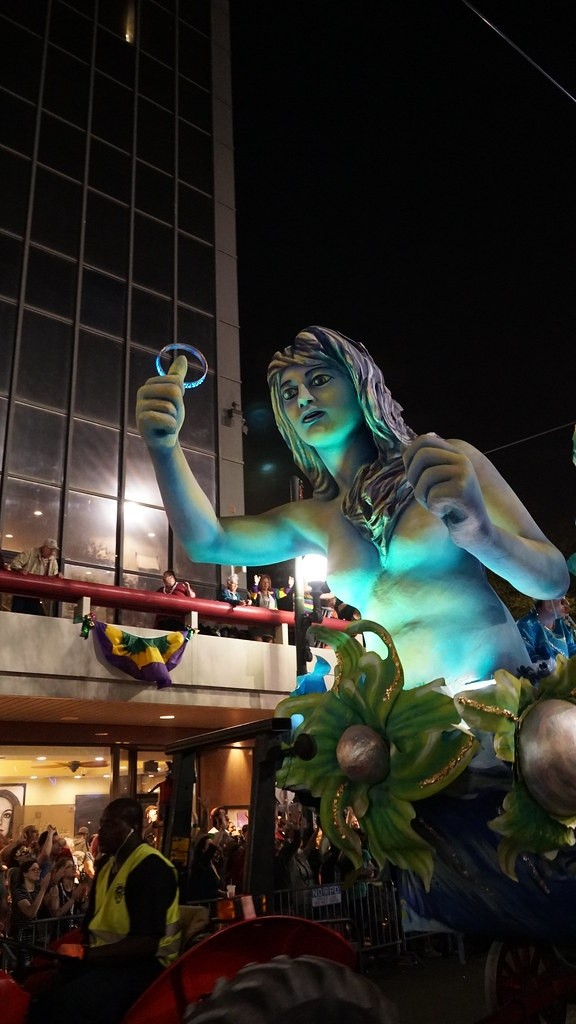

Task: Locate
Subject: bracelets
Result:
[156,343,208,387]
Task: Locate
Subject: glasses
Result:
[65,865,76,869]
[28,869,42,873]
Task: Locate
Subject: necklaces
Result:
[162,582,178,595]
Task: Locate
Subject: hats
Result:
[289,804,301,814]
[43,538,60,550]
[210,807,218,819]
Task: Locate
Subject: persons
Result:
[219,572,361,648]
[0,806,454,983]
[154,569,196,631]
[134,327,576,820]
[9,538,64,616]
[515,596,576,686]
[23,797,181,1024]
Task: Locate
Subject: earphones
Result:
[127,828,135,836]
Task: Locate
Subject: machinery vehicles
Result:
[0,714,402,1024]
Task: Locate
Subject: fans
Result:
[30,761,109,772]
